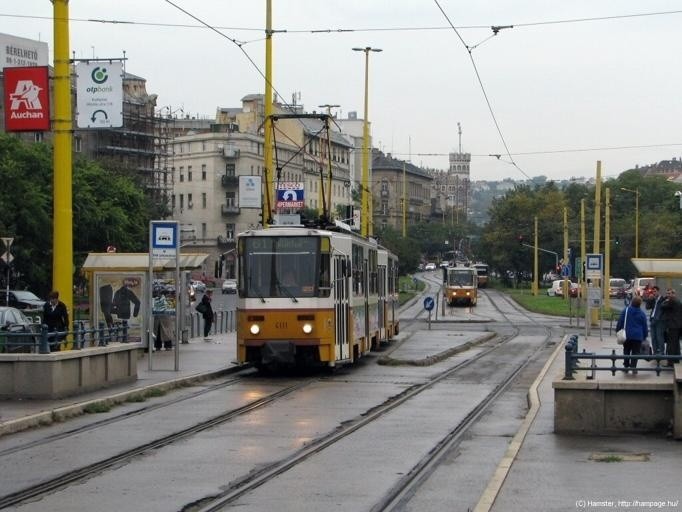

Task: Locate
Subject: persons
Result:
[153,314,172,351]
[195,289,214,339]
[43,292,69,352]
[616,284,682,374]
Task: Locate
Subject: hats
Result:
[48,291,59,298]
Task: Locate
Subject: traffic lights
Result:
[517,233,524,245]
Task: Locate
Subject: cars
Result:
[0,306,39,352]
[502,267,563,282]
[417,260,450,272]
[0,289,47,313]
[150,277,237,311]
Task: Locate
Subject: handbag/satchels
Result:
[195,301,208,313]
[639,340,653,362]
[616,329,627,344]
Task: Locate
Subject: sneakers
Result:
[204,336,212,340]
[621,367,638,374]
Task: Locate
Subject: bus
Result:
[441,266,478,308]
[445,251,462,265]
[468,263,491,288]
[229,218,402,373]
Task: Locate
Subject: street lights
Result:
[619,183,640,279]
[350,46,385,239]
[317,100,341,221]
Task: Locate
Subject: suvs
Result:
[542,276,656,301]
